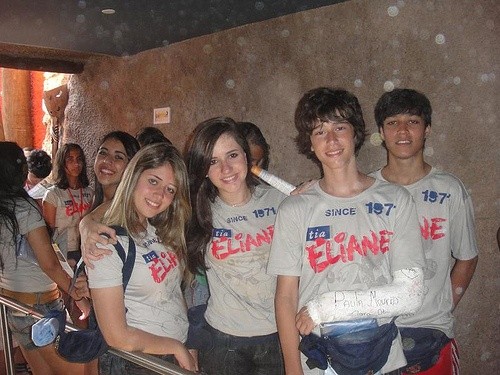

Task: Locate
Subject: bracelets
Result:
[74,296,84,302]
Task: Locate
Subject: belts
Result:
[0,289,59,304]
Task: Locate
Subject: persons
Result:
[0,141,99,374]
[370,88,480,375]
[43,143,96,277]
[83,143,200,375]
[235,121,268,176]
[134,125,171,149]
[23,147,52,197]
[71,127,139,301]
[266,86,424,374]
[79,119,323,375]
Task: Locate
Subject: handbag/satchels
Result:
[299,316,399,375]
[393,325,449,372]
[187,303,209,342]
[55,326,110,363]
